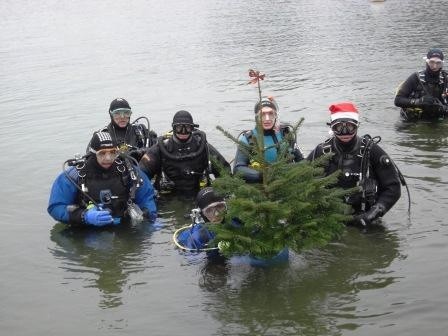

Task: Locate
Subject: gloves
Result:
[433,97,444,112]
[352,203,385,229]
[422,95,433,106]
[84,207,114,226]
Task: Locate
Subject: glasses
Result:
[88,147,117,160]
[201,202,227,217]
[110,110,132,118]
[332,123,357,135]
[427,59,444,67]
[173,124,194,134]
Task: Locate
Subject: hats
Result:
[254,96,279,114]
[427,49,444,61]
[89,130,117,151]
[326,102,359,128]
[109,98,132,113]
[196,187,224,210]
[172,110,194,127]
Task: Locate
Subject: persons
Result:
[47,129,165,250]
[306,103,410,235]
[139,111,232,202]
[394,48,448,132]
[234,96,303,183]
[86,98,163,205]
[175,187,288,292]
